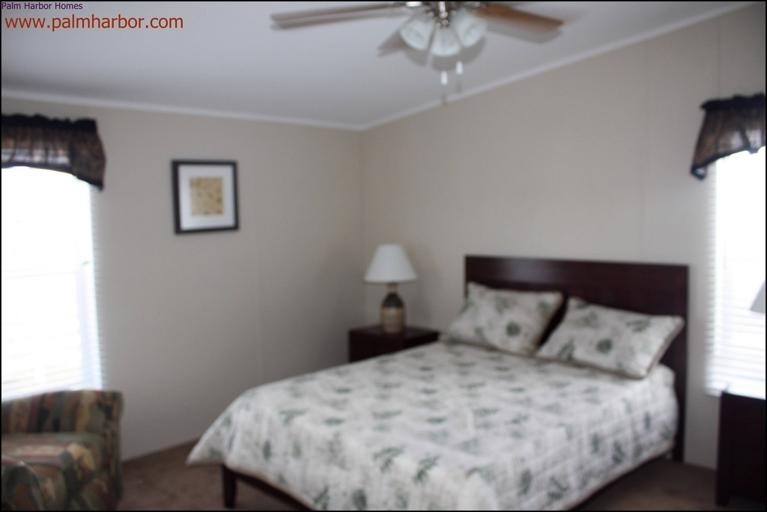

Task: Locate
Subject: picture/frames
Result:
[172,160,239,234]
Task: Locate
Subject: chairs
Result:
[2,390,123,510]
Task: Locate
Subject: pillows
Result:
[533,297,685,379]
[439,283,564,357]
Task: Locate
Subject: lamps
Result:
[362,243,418,336]
[400,2,487,58]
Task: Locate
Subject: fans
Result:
[271,1,564,52]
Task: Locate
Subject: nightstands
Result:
[714,381,766,507]
[348,324,440,362]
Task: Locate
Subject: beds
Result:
[186,256,688,511]
